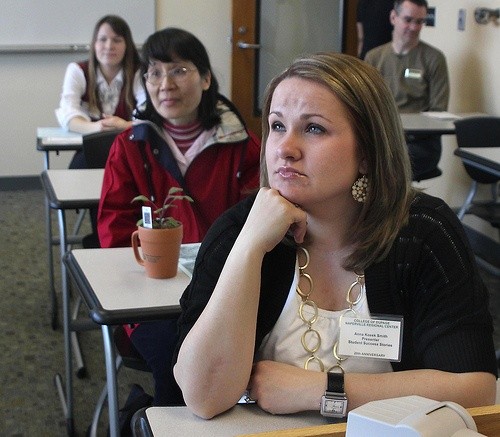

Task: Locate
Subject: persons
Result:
[55,15,183,170]
[98,28,261,407]
[362,0,450,173]
[173,52,498,420]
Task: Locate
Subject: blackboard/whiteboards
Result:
[0,0,157,54]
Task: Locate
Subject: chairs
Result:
[80,127,154,437]
[453,115,500,278]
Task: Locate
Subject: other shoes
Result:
[119,383,153,437]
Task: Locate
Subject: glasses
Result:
[143,66,199,85]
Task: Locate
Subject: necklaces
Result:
[294,246,369,372]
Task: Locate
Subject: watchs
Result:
[320,372,349,424]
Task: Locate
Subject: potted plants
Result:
[130,186,194,280]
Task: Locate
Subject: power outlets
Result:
[458,9,466,31]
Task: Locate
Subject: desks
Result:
[35,112,500,437]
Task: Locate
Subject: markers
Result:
[70,45,89,49]
